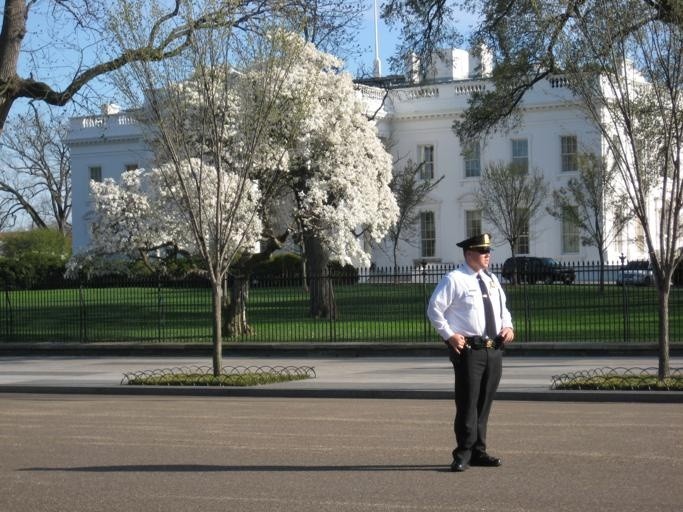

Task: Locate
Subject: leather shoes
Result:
[450,452,501,472]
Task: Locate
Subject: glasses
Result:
[479,250,490,254]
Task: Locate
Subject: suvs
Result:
[501,256,576,285]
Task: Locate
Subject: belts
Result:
[465,337,504,349]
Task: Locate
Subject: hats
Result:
[456,233,494,251]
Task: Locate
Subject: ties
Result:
[476,274,497,340]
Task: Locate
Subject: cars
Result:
[618,259,665,285]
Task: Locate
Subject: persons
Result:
[424,232,514,471]
